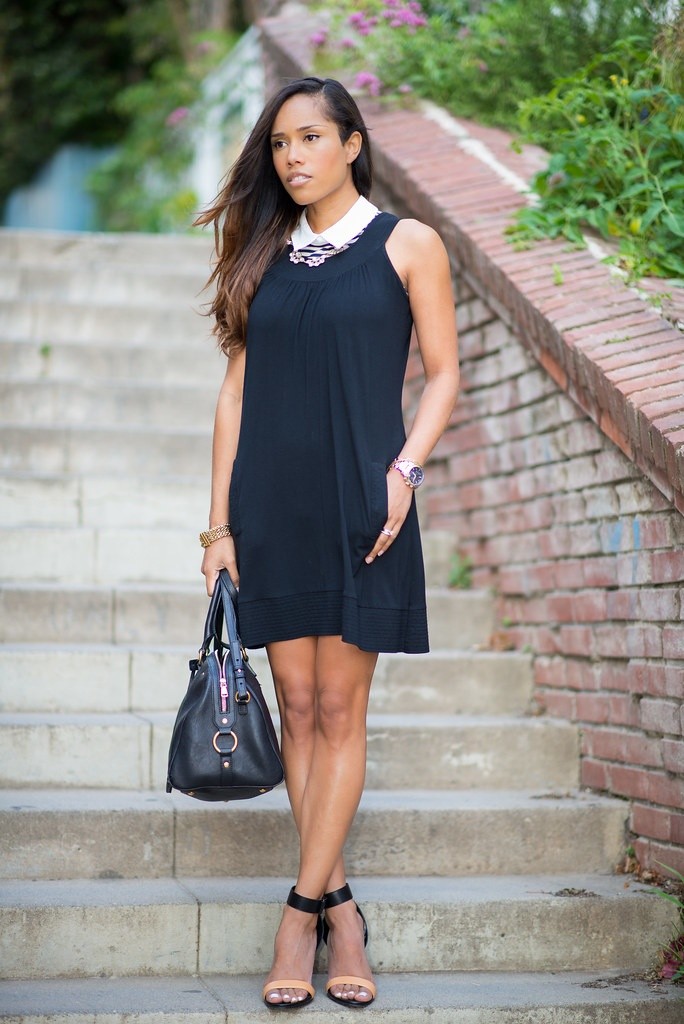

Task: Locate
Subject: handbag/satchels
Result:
[166,569,286,801]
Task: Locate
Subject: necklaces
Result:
[287,239,345,268]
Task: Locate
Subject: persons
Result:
[200,77,461,1010]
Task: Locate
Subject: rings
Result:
[381,528,392,536]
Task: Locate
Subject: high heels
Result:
[318,882,376,1008]
[262,885,322,1009]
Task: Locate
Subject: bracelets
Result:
[199,522,231,549]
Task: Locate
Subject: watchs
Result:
[386,458,424,489]
